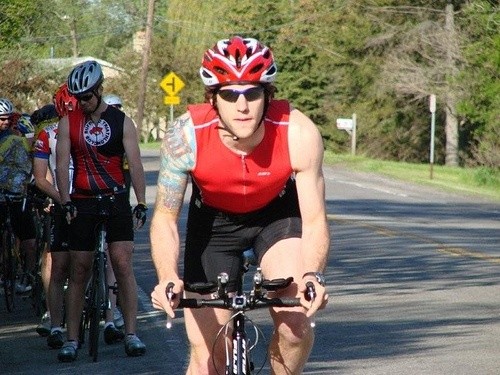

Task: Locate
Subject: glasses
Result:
[74,93,95,104]
[0,117,12,121]
[213,86,267,103]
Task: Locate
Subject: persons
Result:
[150,36,330,375]
[57,60,148,361]
[0,83,132,347]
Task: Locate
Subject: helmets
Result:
[0,98,13,115]
[52,82,77,118]
[102,92,123,106]
[200,34,278,87]
[11,112,35,134]
[66,61,106,95]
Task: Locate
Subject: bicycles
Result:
[0,193,141,362]
[166,247,318,375]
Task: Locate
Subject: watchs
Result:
[302,272,327,286]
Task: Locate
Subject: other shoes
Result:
[113,305,124,327]
[103,324,124,345]
[47,328,65,349]
[36,311,52,335]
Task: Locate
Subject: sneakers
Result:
[124,333,147,357]
[57,339,82,364]
